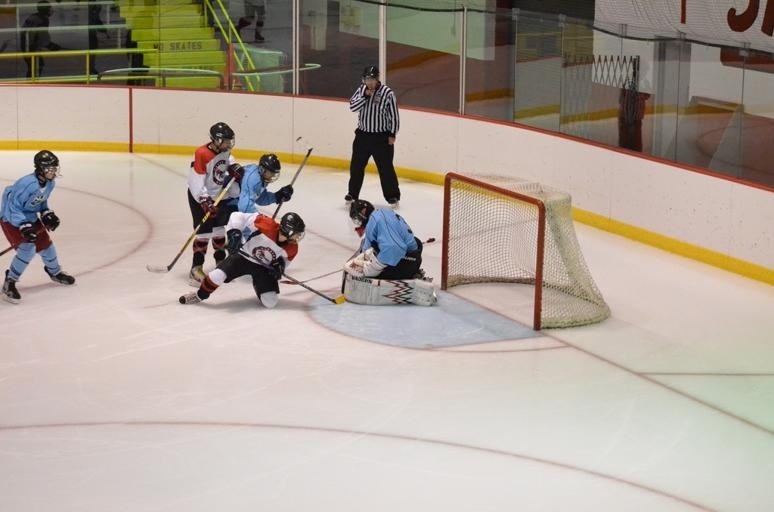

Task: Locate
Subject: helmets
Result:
[280,213,305,244]
[210,123,235,152]
[259,154,280,183]
[350,200,374,225]
[34,150,62,181]
[360,66,379,84]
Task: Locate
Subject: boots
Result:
[3,269,20,299]
[43,265,74,284]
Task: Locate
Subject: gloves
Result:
[275,185,293,204]
[269,256,284,280]
[41,208,60,231]
[19,221,37,241]
[229,163,244,183]
[227,229,242,255]
[199,196,219,218]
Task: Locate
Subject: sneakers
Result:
[388,197,397,204]
[345,192,352,201]
[179,290,210,304]
[191,265,206,282]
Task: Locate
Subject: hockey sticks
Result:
[271,137,312,220]
[223,244,346,304]
[146,176,234,274]
[280,238,436,285]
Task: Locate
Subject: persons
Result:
[234,0,266,42]
[1,151,75,304]
[124,1,160,85]
[615,58,652,153]
[225,154,293,241]
[179,211,306,308]
[338,65,400,210]
[344,200,437,308]
[87,0,108,74]
[20,2,62,79]
[187,122,235,285]
[193,1,221,32]
